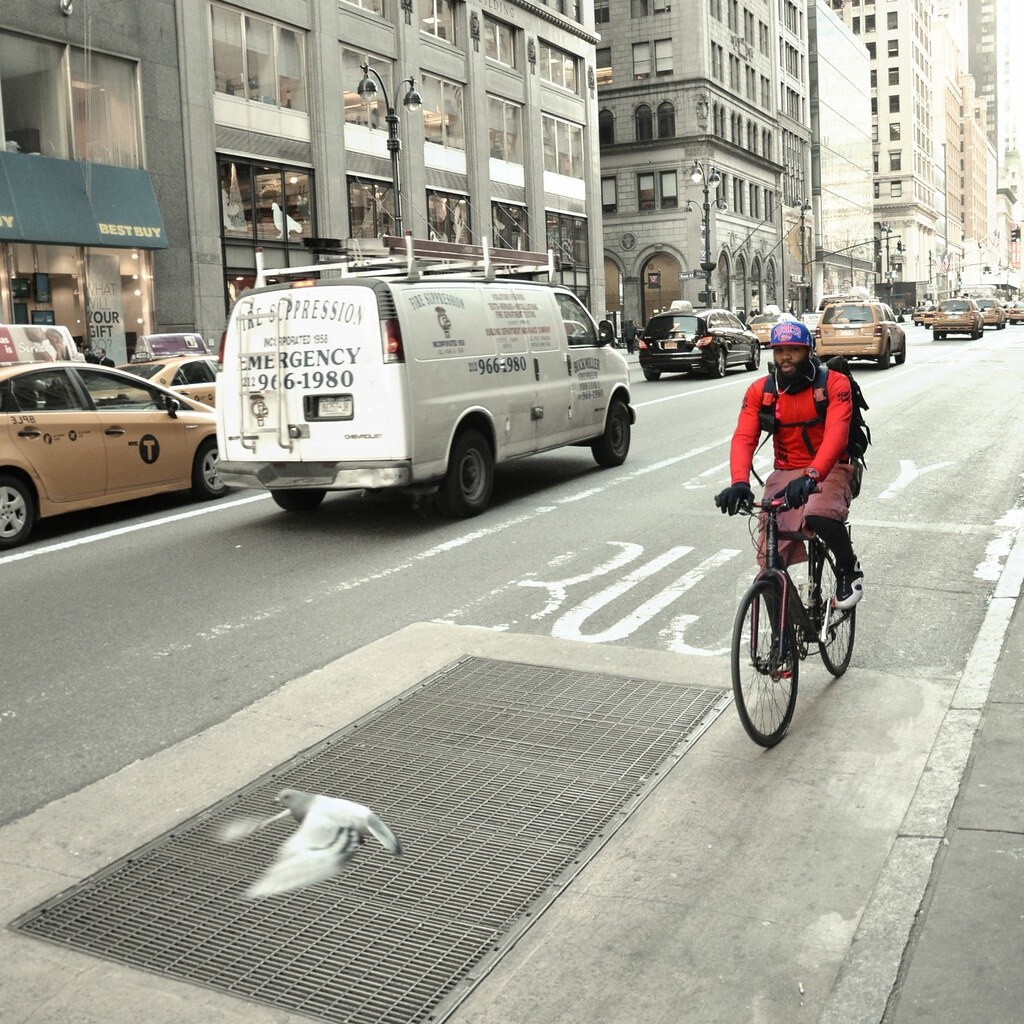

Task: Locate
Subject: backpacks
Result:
[759,356,871,457]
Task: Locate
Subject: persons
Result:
[46,329,70,360]
[716,322,863,672]
[746,309,760,325]
[623,320,637,354]
[894,303,901,316]
[790,307,794,315]
[96,348,115,367]
[25,327,63,361]
[738,311,745,325]
[83,346,99,363]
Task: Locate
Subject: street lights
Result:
[357,62,423,237]
[794,195,811,313]
[684,159,728,310]
[882,222,893,307]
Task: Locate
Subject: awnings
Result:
[0,151,170,250]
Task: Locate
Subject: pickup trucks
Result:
[801,294,852,337]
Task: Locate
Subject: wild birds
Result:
[270,202,303,240]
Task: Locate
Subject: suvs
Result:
[638,309,761,382]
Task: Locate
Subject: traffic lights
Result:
[983,266,992,275]
[1011,228,1021,243]
[897,241,906,254]
[713,291,718,302]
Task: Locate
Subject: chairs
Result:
[185,364,213,383]
[13,380,72,409]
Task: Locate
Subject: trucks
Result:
[214,231,636,518]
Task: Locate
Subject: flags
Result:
[936,249,953,273]
[988,229,1000,248]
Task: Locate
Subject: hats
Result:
[766,321,813,348]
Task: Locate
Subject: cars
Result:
[816,302,906,369]
[0,322,230,550]
[88,333,218,408]
[912,296,1024,340]
[749,293,800,349]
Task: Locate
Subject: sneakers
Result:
[760,632,794,671]
[833,556,864,608]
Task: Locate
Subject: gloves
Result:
[715,482,755,516]
[774,474,818,510]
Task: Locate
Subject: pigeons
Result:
[215,786,405,903]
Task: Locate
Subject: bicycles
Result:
[714,485,857,748]
